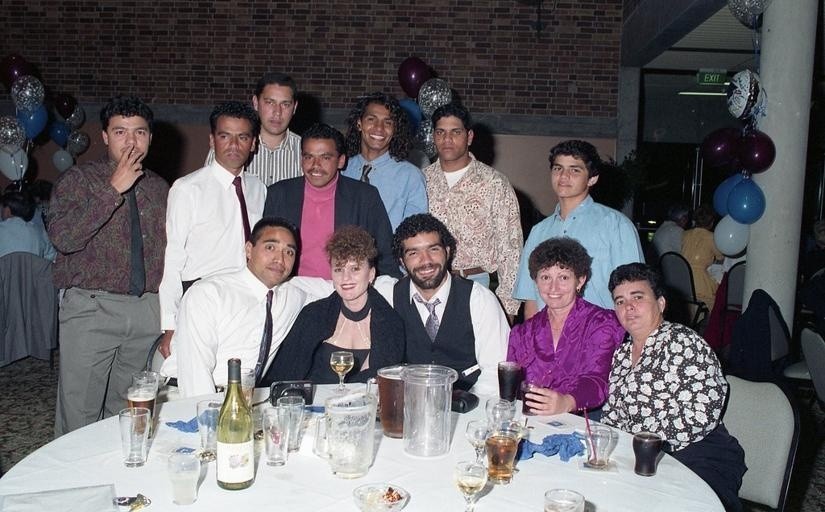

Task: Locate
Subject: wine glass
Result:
[330,349,354,397]
[453,420,492,512]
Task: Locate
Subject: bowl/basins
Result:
[352,481,410,512]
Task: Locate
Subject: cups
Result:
[486,359,521,422]
[400,364,457,457]
[237,367,304,466]
[118,368,161,466]
[167,398,222,506]
[583,421,663,479]
[485,430,517,485]
[544,489,587,512]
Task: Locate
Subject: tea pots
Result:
[366,361,408,438]
[313,391,379,479]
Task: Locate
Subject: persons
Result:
[47,94,169,436]
[0,192,58,262]
[155,90,654,420]
[34,179,55,207]
[4,178,31,192]
[598,261,750,512]
[648,201,824,336]
[201,73,303,192]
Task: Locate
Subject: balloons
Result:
[0,46,90,186]
[393,53,452,160]
[705,2,778,255]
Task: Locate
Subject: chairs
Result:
[145,334,174,388]
[718,259,745,345]
[729,287,817,409]
[720,374,812,512]
[656,251,710,330]
[799,325,825,412]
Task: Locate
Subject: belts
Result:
[449,266,485,277]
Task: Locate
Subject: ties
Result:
[414,293,441,344]
[232,176,251,244]
[254,290,275,389]
[360,164,372,185]
[129,187,145,297]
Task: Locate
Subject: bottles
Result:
[213,358,256,490]
